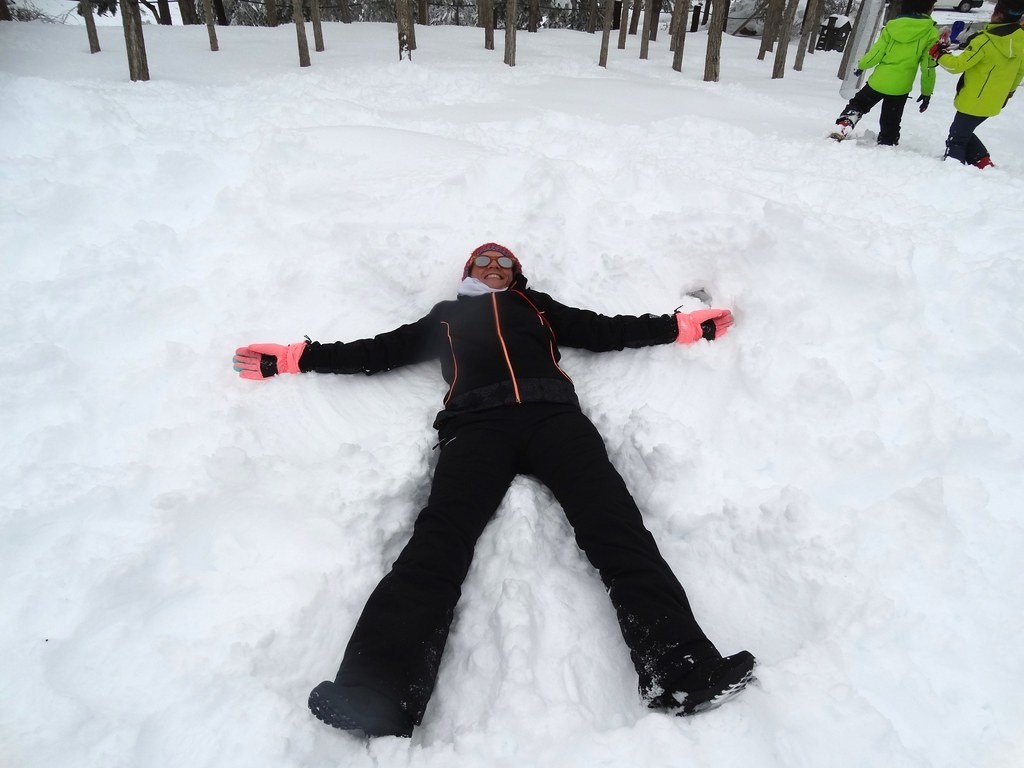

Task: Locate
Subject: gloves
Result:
[232,342,307,380]
[929,42,949,61]
[917,94,930,113]
[854,68,863,77]
[675,309,734,344]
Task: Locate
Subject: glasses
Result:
[471,255,514,269]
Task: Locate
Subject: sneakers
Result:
[638,651,754,717]
[976,156,994,169]
[309,681,414,738]
[827,120,849,142]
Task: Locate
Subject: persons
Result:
[929,0,1024,169]
[830,0,940,146]
[231,241,758,739]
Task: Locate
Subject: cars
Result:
[934,0,982,12]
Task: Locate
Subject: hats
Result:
[462,243,522,281]
